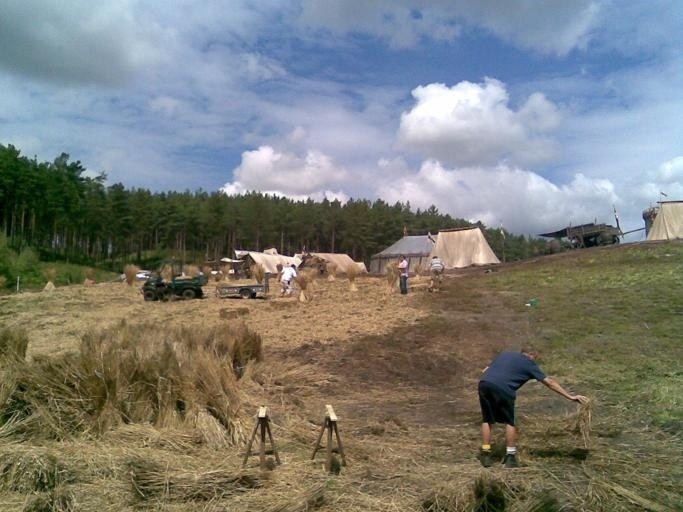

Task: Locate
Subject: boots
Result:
[478,452,491,467]
[505,454,528,468]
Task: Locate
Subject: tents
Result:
[646,201,683,240]
[425,225,501,270]
[369,235,435,273]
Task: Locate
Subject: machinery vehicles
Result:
[142,257,207,302]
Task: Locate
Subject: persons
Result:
[397,255,408,294]
[479,346,587,467]
[276,264,297,297]
[429,255,444,292]
